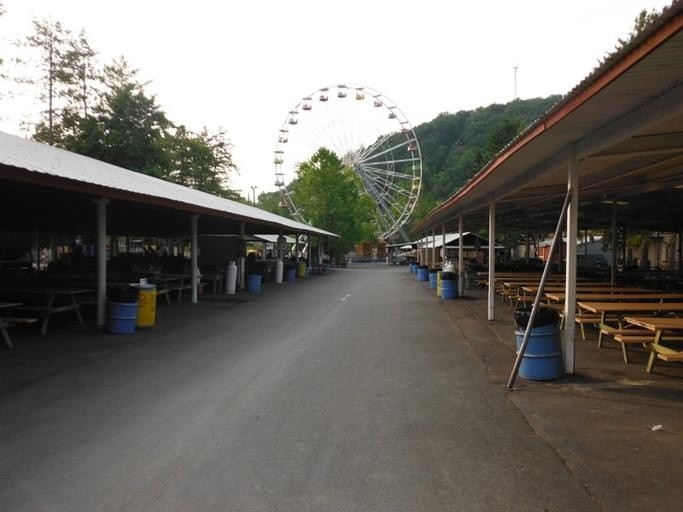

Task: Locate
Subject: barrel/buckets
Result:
[284,261,305,282]
[131,278,157,327]
[247,273,262,293]
[409,261,458,299]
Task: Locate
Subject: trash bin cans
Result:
[408,262,458,299]
[284,267,295,282]
[246,272,262,293]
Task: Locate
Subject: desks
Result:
[476,271,683,374]
